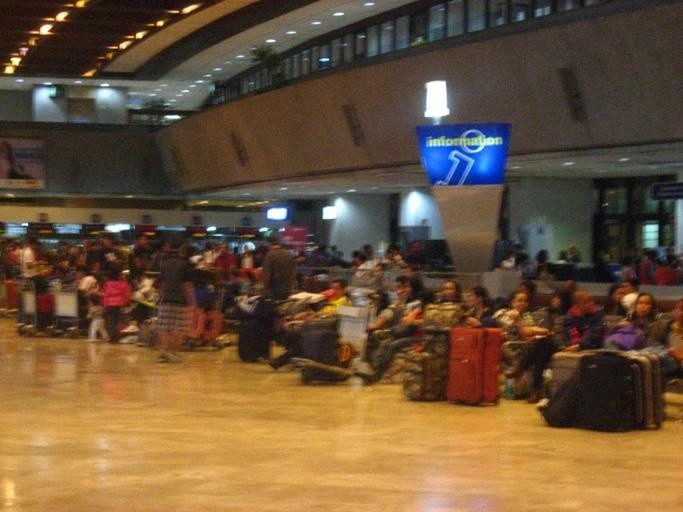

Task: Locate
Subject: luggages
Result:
[238,318,348,385]
[402,324,505,405]
[538,349,666,432]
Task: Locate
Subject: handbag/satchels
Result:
[103,280,133,307]
[233,294,277,321]
[424,302,465,333]
[562,300,604,349]
[603,324,646,351]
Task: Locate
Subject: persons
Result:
[350,241,683,411]
[192,234,373,369]
[1,229,196,363]
